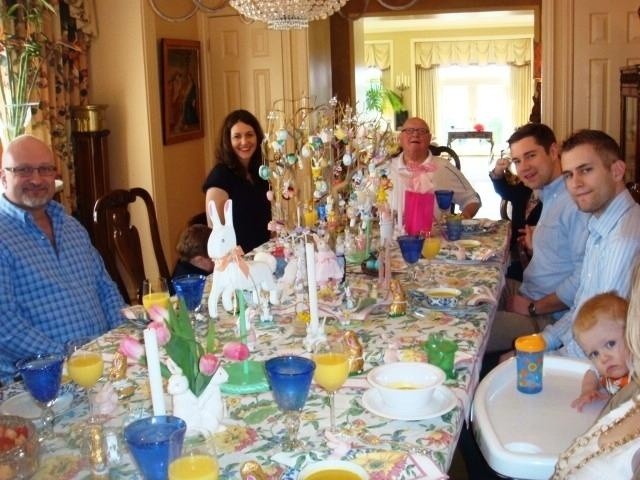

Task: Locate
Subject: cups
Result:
[168,427,218,480]
[368,361,445,407]
[424,339,458,376]
[123,416,190,480]
[142,278,170,316]
[0,416,38,480]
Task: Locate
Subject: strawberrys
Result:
[0,423,27,452]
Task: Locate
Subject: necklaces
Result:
[551,400,640,479]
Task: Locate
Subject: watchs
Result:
[528,301,538,316]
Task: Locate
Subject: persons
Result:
[167,223,214,298]
[569,289,630,412]
[459,129,640,479]
[488,156,544,281]
[382,117,482,218]
[0,134,133,395]
[201,109,278,256]
[481,123,591,351]
[549,248,640,480]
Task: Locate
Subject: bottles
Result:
[86,427,110,480]
[515,332,547,395]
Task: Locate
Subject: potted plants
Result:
[0,0,57,153]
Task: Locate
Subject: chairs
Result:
[91,187,174,308]
[396,144,461,216]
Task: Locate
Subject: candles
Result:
[396,184,405,230]
[305,242,320,324]
[143,328,167,415]
[394,71,410,89]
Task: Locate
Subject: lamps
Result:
[228,0,351,32]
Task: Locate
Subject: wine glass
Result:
[313,341,356,438]
[174,276,209,342]
[265,356,315,454]
[272,180,501,317]
[13,353,67,443]
[67,336,108,430]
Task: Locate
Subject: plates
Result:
[0,391,74,420]
[358,388,461,422]
[297,460,368,479]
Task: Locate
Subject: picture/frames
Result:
[162,37,204,147]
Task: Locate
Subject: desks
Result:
[446,131,494,156]
[471,347,614,480]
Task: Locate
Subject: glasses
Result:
[3,164,58,177]
[401,127,429,135]
